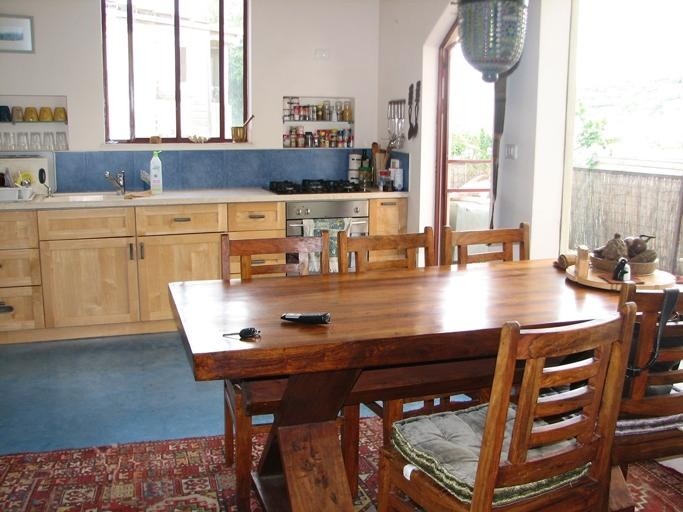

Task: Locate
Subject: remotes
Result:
[280,312,331,325]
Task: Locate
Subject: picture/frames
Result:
[0,13,36,53]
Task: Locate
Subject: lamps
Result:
[456,0,527,83]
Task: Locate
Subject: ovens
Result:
[286,219,368,274]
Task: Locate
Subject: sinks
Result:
[37,192,126,204]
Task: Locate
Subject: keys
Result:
[222,327,259,339]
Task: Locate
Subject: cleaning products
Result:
[150,150,162,195]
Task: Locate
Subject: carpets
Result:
[2,417,680,509]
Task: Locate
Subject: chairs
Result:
[377,302,637,511]
[219,228,360,510]
[528,281,682,473]
[336,227,476,492]
[440,221,552,407]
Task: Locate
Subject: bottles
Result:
[293,101,351,121]
[150,152,162,193]
[290,129,353,149]
[377,170,393,191]
[360,149,369,168]
[149,120,161,144]
[675,258,683,284]
[575,247,589,277]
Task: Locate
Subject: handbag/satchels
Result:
[572,292,680,407]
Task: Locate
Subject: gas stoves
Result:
[263,178,370,219]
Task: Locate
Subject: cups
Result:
[0,106,66,123]
[0,131,69,152]
[231,127,245,143]
[19,188,33,200]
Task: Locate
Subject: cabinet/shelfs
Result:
[368,198,409,270]
[37,204,229,340]
[281,96,354,148]
[0,94,67,151]
[0,209,46,343]
[228,202,287,278]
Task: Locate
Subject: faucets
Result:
[104,169,126,194]
[43,184,53,197]
